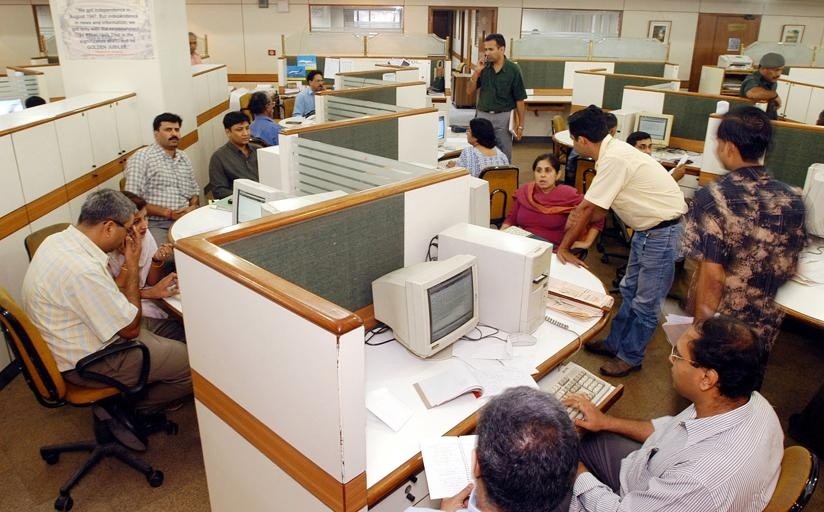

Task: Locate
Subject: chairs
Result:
[763,447,820,512]
[240,93,284,124]
[248,138,270,150]
[25,223,73,262]
[480,166,519,229]
[283,97,295,117]
[601,213,685,300]
[551,116,568,165]
[574,155,595,195]
[0,288,178,512]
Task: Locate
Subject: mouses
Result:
[506,333,538,346]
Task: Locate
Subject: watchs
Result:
[772,94,778,99]
[518,126,524,130]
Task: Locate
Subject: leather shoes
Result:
[93,410,167,445]
[91,402,149,452]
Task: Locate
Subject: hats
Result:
[759,52,786,68]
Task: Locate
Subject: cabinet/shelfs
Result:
[451,72,475,108]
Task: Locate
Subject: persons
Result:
[557,106,690,377]
[291,70,326,119]
[564,113,618,186]
[105,190,178,341]
[562,317,785,512]
[122,113,201,271]
[691,105,804,393]
[448,118,509,178]
[500,154,606,253]
[440,386,579,512]
[741,52,785,121]
[245,92,285,145]
[627,132,686,184]
[209,112,272,202]
[467,34,528,166]
[23,190,194,435]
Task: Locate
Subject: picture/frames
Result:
[648,21,672,45]
[780,25,806,45]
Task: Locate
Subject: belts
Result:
[478,108,511,115]
[647,218,680,231]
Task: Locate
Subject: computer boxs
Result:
[438,222,553,335]
[801,163,822,238]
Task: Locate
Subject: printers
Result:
[718,54,752,71]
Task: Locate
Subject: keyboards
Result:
[545,361,615,419]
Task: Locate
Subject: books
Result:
[415,368,539,408]
[420,435,476,500]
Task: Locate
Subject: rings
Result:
[163,255,166,256]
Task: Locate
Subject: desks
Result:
[553,129,575,148]
[367,226,614,512]
[651,147,703,199]
[516,89,572,143]
[698,65,755,97]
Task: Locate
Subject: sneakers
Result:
[599,355,642,377]
[583,338,618,359]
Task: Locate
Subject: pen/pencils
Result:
[166,283,176,291]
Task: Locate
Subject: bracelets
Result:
[152,261,166,270]
[120,267,129,271]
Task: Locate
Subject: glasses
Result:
[669,344,696,364]
[103,219,134,236]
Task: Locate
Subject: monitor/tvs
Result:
[634,111,674,153]
[372,253,480,359]
[438,112,449,148]
[232,178,282,225]
